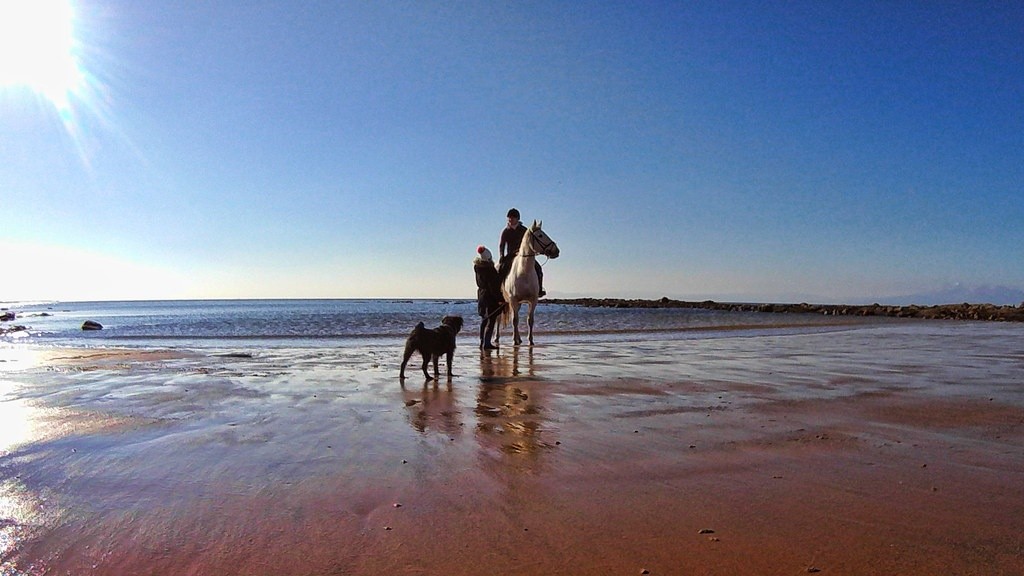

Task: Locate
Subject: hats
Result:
[507,209,520,220]
[478,247,492,260]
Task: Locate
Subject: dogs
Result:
[399,313,464,380]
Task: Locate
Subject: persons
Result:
[473,245,505,350]
[498,208,546,302]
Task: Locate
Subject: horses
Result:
[497,218,562,344]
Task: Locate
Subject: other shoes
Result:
[480,326,485,346]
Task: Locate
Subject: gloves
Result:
[499,256,504,263]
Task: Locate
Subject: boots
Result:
[483,327,500,349]
[538,276,546,297]
